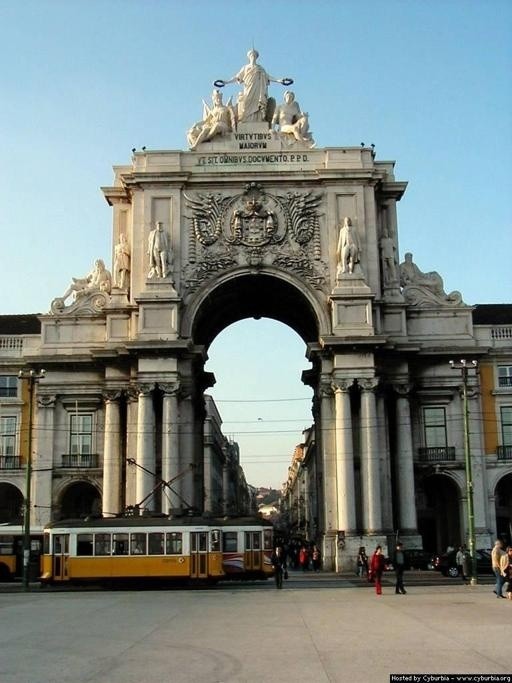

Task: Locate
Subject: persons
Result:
[490,539,507,598]
[446,542,455,552]
[368,544,390,594]
[223,49,284,122]
[456,546,470,581]
[146,220,174,278]
[379,227,400,283]
[270,91,311,143]
[501,545,512,599]
[114,232,130,289]
[334,215,363,273]
[60,258,112,303]
[400,252,447,297]
[270,545,288,590]
[392,542,408,593]
[187,89,238,150]
[270,533,322,573]
[358,547,371,576]
[134,544,143,554]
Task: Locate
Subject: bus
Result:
[0,505,276,588]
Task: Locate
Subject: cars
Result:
[431,549,496,578]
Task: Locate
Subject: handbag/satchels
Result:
[367,570,375,584]
[284,569,289,580]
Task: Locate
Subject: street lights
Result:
[19,369,46,591]
[449,359,478,586]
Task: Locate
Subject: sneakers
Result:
[395,590,407,594]
[276,583,282,589]
[493,590,511,600]
